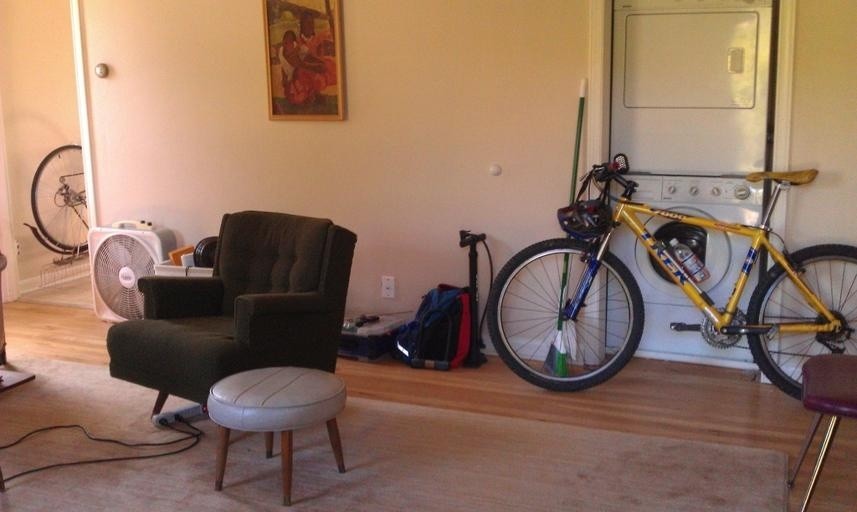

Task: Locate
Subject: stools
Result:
[206,366,347,507]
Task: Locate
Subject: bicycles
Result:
[485,163,857,404]
[22,144,89,267]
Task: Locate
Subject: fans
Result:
[88,220,174,324]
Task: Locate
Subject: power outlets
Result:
[381,276,394,298]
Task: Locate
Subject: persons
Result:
[280,10,336,106]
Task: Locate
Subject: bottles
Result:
[668,238,710,283]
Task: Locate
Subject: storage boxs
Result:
[154,259,214,277]
[337,318,404,362]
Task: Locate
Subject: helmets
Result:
[556,197,613,240]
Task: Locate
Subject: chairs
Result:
[106,210,356,420]
[789,355,857,512]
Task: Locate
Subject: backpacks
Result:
[392,279,487,372]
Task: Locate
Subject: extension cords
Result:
[152,404,207,427]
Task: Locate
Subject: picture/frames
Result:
[262,0,346,122]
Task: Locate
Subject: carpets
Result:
[0,357,790,512]
